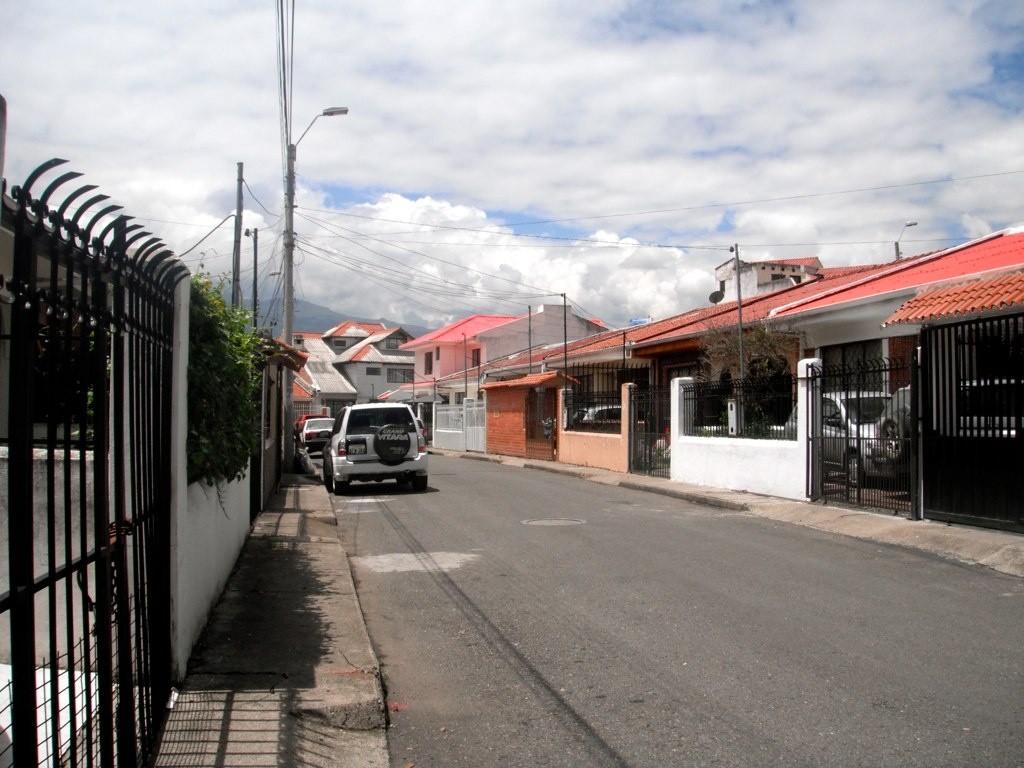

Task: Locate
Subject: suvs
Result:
[573,405,622,421]
[784,376,1023,487]
[322,402,429,495]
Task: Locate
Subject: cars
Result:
[296,415,338,451]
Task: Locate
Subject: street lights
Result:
[893,220,919,260]
[284,106,348,471]
